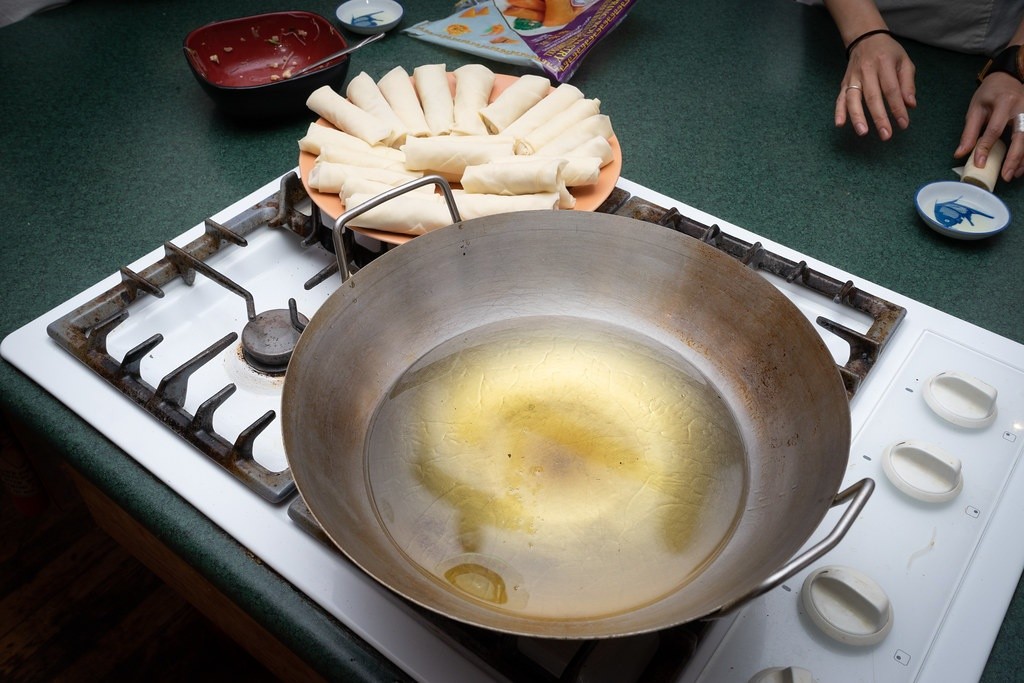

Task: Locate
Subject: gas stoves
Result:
[44,160,914,681]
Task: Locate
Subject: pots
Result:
[281,175,879,644]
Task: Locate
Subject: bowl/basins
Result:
[181,12,353,125]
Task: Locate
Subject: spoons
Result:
[286,29,384,80]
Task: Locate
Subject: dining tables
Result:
[2,1,1024,683]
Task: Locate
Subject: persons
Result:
[797,0,1024,185]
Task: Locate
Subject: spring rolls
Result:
[299,64,614,235]
[504,1,577,26]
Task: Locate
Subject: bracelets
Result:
[845,30,896,60]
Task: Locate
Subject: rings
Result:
[1009,112,1023,133]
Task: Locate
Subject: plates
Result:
[301,70,623,244]
[913,180,1012,239]
[334,0,404,36]
